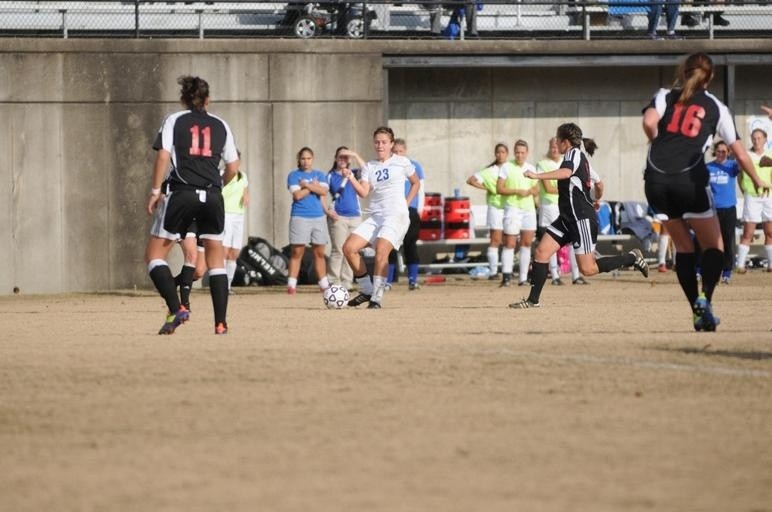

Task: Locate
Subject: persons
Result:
[443,0,480,40]
[681,0,730,26]
[696,141,744,286]
[646,206,676,272]
[648,0,683,41]
[174,220,208,312]
[144,77,239,335]
[535,137,590,286]
[737,128,772,275]
[384,138,425,290]
[343,126,420,309]
[496,140,540,287]
[641,53,770,331]
[220,149,248,295]
[466,144,520,281]
[320,146,366,292]
[509,123,649,309]
[286,147,329,293]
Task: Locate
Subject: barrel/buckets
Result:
[419,191,443,240]
[443,197,471,240]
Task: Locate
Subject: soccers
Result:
[322,284,349,309]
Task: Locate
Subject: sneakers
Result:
[572,277,590,284]
[214,320,228,334]
[720,275,730,285]
[158,305,191,336]
[629,247,650,279]
[693,293,717,332]
[693,313,721,332]
[508,299,541,309]
[656,264,666,272]
[367,301,382,309]
[408,281,421,290]
[549,278,562,286]
[347,292,372,307]
[499,272,511,288]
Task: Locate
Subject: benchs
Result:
[563,6,726,40]
[396,235,633,276]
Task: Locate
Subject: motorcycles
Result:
[290,0,376,40]
